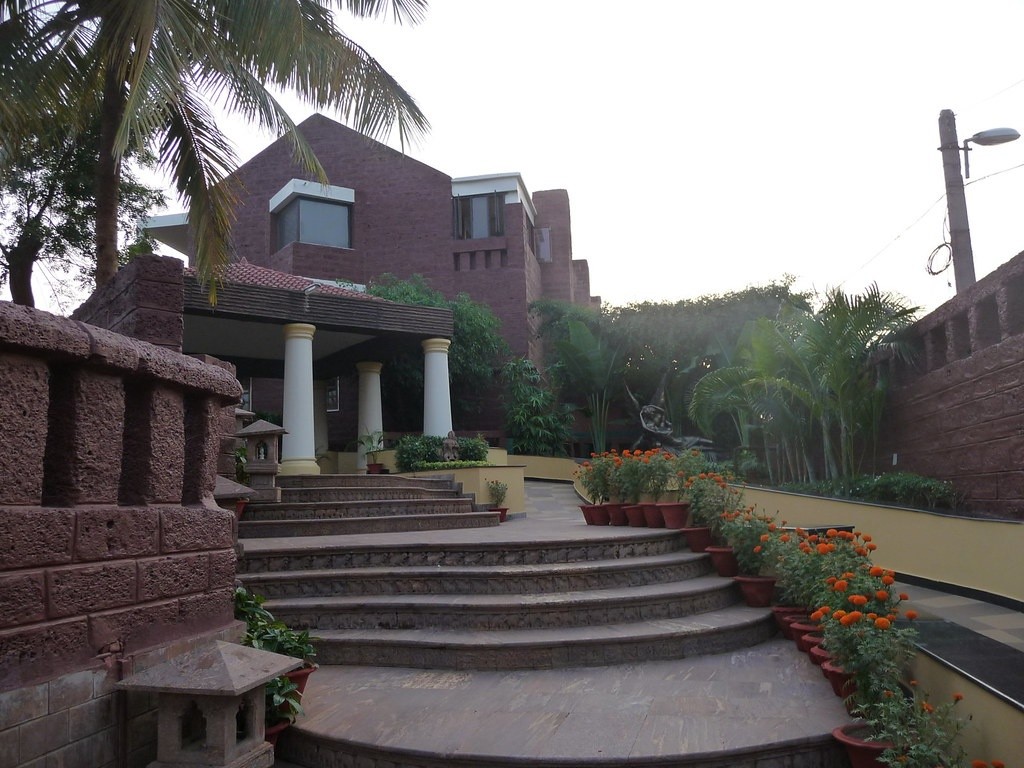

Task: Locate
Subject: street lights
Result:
[938,109,1021,294]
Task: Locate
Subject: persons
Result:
[634,404,681,456]
[442,432,459,462]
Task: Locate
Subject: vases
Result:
[217,497,249,521]
[577,502,718,554]
[832,721,919,768]
[735,575,781,607]
[488,507,510,523]
[770,604,877,718]
[705,545,740,577]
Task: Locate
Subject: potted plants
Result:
[345,423,392,475]
[232,581,318,752]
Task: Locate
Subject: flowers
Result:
[880,674,1010,768]
[821,564,914,602]
[719,503,772,575]
[809,607,927,721]
[486,476,512,507]
[752,512,883,604]
[574,443,760,498]
